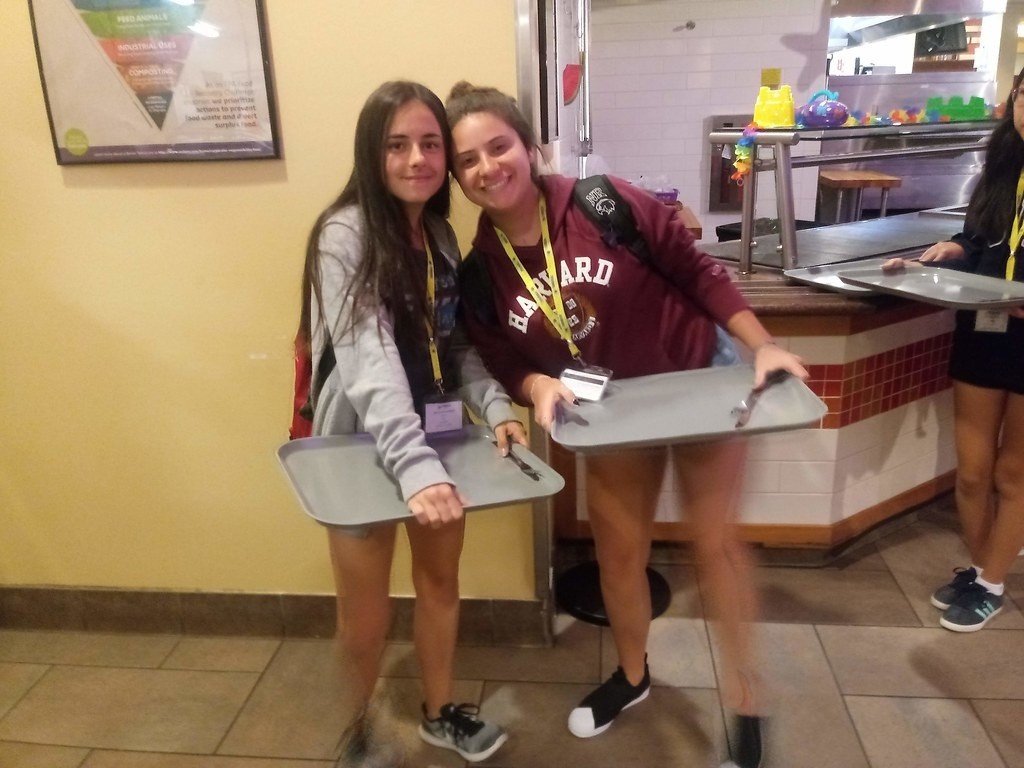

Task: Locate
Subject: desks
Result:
[674,206,704,241]
[818,169,903,226]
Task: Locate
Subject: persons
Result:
[288,77,530,768]
[442,79,812,768]
[882,66,1024,631]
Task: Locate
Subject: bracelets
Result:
[754,341,776,357]
[530,375,552,404]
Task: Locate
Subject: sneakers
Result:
[418,701,508,762]
[569,653,650,739]
[931,566,977,609]
[940,583,1004,633]
[737,717,764,768]
